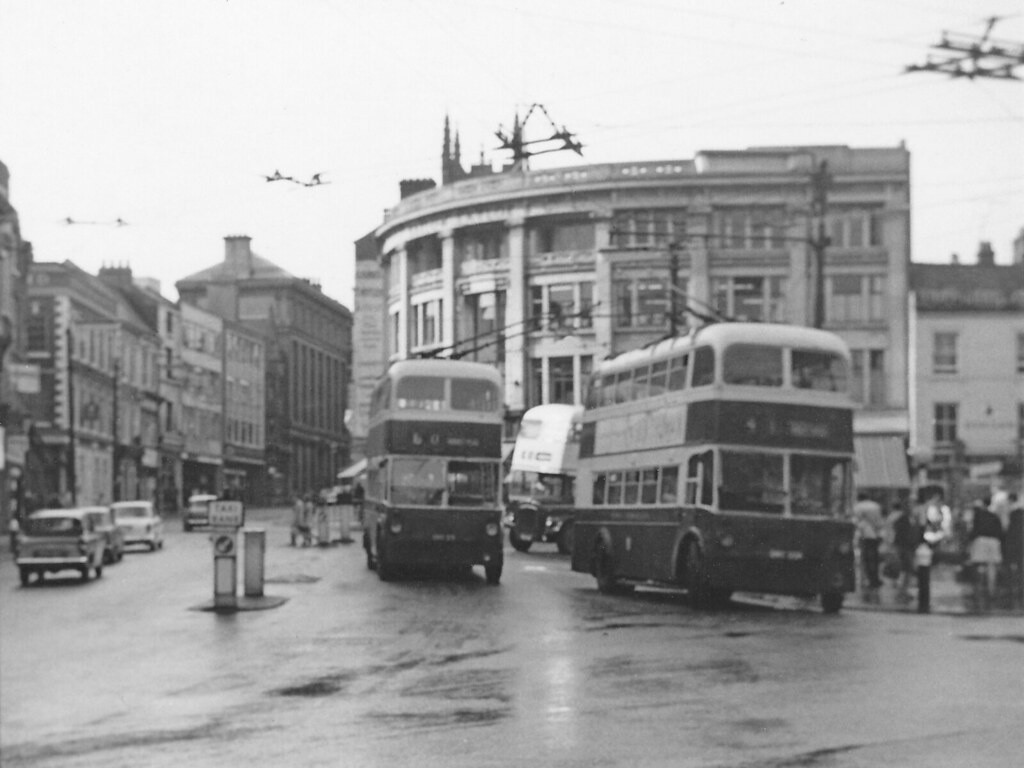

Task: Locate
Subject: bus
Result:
[571,321,858,618]
[359,357,506,584]
[502,404,589,561]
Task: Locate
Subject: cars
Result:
[15,509,107,581]
[78,506,125,564]
[183,493,220,531]
[113,501,164,554]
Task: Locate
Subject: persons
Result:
[852,491,1023,608]
